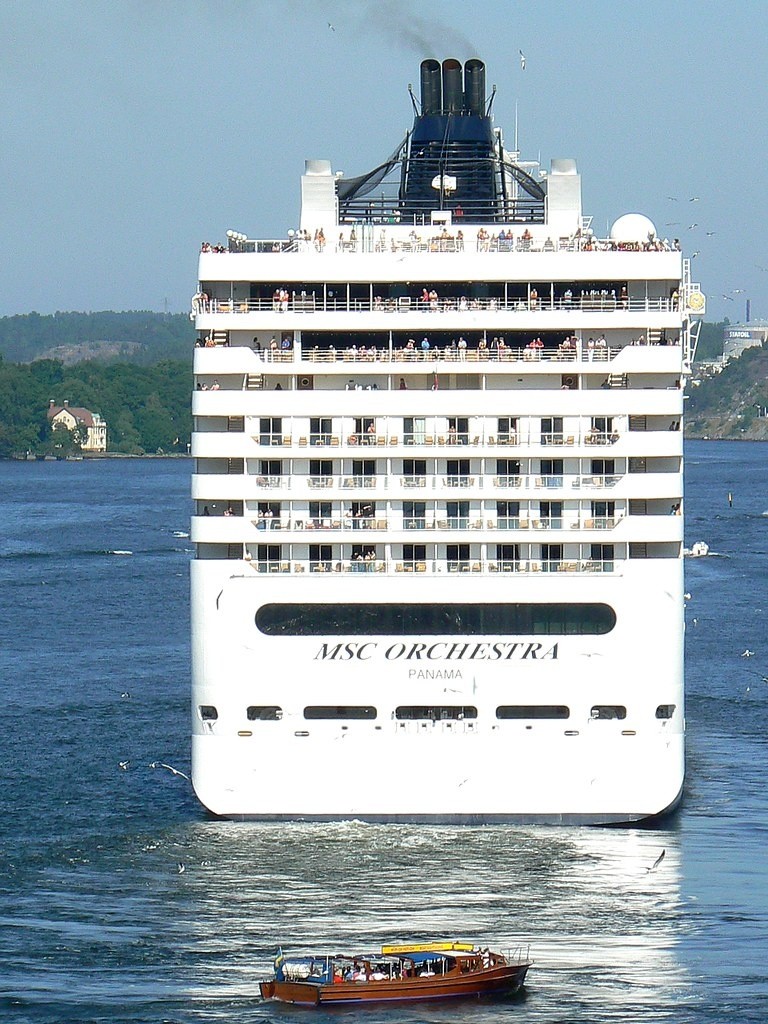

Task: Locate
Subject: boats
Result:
[257,938,536,1013]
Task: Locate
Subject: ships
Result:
[188,56,706,835]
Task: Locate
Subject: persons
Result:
[351,550,375,572]
[369,962,401,980]
[610,429,619,445]
[197,380,219,390]
[196,229,680,363]
[561,381,569,390]
[202,505,209,516]
[246,549,251,561]
[368,423,376,445]
[669,421,680,431]
[450,426,456,445]
[601,379,610,389]
[399,378,407,390]
[333,964,366,983]
[372,384,379,390]
[453,204,464,222]
[224,507,234,516]
[258,509,273,529]
[345,504,374,529]
[675,380,680,388]
[403,959,441,978]
[275,383,282,390]
[391,209,400,223]
[590,426,600,444]
[511,426,516,445]
[670,504,680,515]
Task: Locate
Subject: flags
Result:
[274,949,285,972]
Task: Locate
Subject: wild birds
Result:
[664,197,679,203]
[327,22,336,31]
[148,760,160,771]
[687,223,700,231]
[121,692,130,702]
[706,231,716,237]
[640,850,666,873]
[177,862,186,877]
[730,289,747,294]
[519,49,528,71]
[739,650,756,658]
[688,250,701,258]
[665,222,679,226]
[688,197,700,203]
[159,763,190,781]
[722,293,734,301]
[117,760,129,770]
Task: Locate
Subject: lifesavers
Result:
[301,377,310,387]
[690,293,704,309]
[281,963,311,979]
[565,376,574,386]
